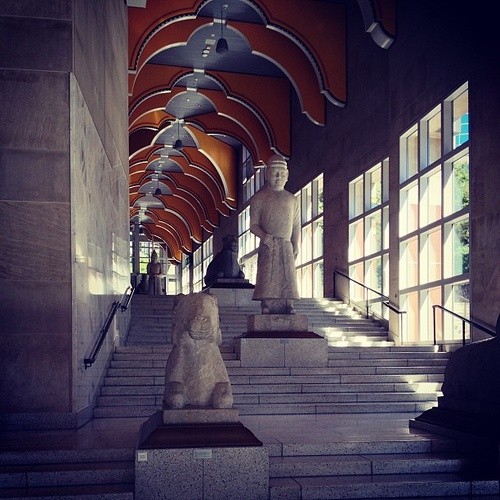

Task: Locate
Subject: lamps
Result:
[155,175,162,196]
[174,121,184,150]
[216,10,229,54]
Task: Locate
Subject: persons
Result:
[249,154,302,313]
[147,250,164,296]
